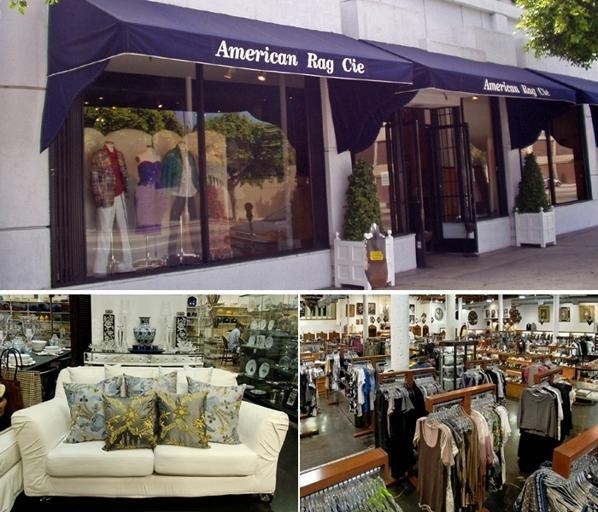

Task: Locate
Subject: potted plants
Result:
[329,157,398,289]
[513,147,557,249]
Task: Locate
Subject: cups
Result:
[132,344,158,351]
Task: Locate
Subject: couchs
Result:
[7,358,291,507]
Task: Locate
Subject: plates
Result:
[246,335,274,349]
[250,389,268,395]
[245,385,255,390]
[245,360,257,375]
[249,319,277,331]
[258,361,271,378]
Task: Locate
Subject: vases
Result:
[133,316,158,348]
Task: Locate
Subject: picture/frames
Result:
[577,302,595,325]
[536,305,551,325]
[558,306,572,324]
[345,302,376,318]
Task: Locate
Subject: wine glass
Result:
[88,339,117,354]
[0,313,35,352]
[159,343,195,355]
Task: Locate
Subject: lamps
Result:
[256,69,267,81]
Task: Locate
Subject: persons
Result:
[90,141,136,275]
[132,144,168,235]
[228,322,244,365]
[164,142,202,263]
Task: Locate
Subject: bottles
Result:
[40,315,44,321]
[44,315,50,321]
[279,390,286,405]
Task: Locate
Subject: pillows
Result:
[153,389,214,451]
[58,374,126,446]
[123,370,181,401]
[184,373,246,447]
[100,392,158,451]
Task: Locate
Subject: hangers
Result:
[300,342,595,512]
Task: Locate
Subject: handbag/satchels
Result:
[3,348,42,409]
[0,349,23,414]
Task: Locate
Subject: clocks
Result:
[434,308,444,321]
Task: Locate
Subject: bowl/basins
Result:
[43,345,62,354]
[32,340,47,351]
[8,354,34,364]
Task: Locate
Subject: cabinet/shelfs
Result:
[0,299,69,350]
[186,304,208,357]
[214,305,251,352]
